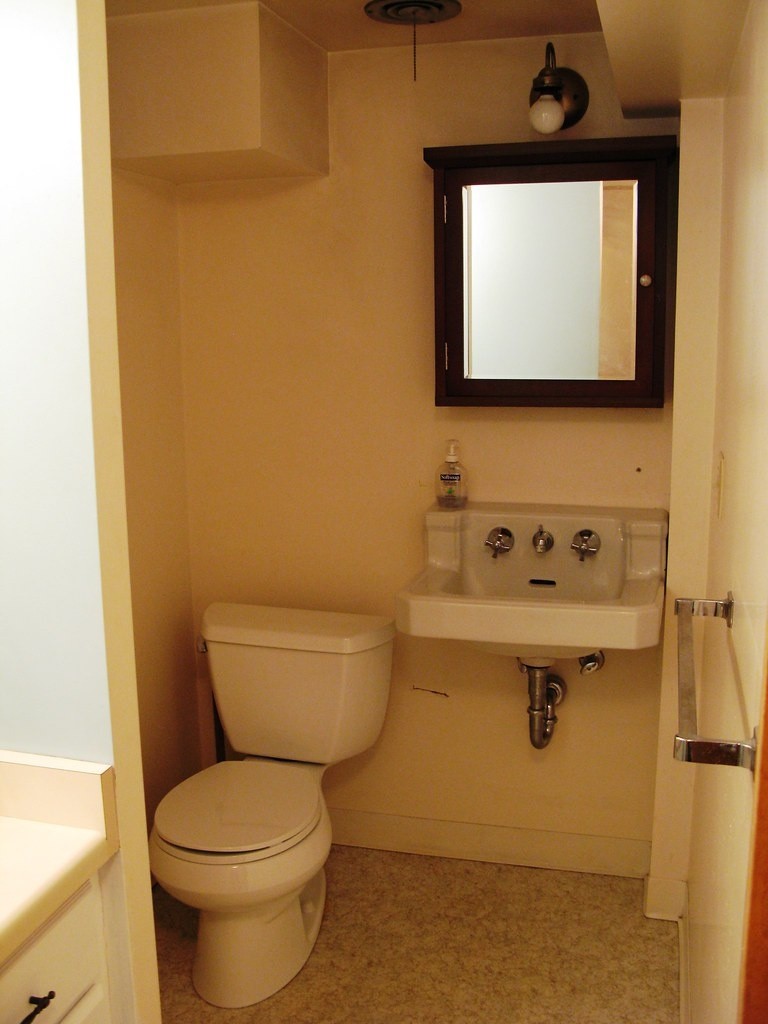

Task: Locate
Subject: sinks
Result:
[395,565,666,659]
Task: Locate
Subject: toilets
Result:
[148,600,395,1009]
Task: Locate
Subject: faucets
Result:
[532,531,554,554]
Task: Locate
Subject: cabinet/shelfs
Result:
[1,869,111,1023]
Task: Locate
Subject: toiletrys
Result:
[435,439,468,509]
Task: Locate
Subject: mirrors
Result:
[422,130,680,416]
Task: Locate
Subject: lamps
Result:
[528,42,590,134]
